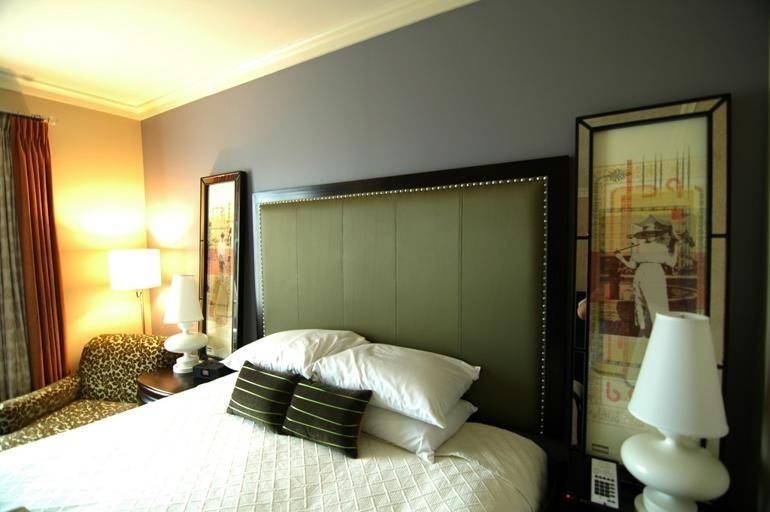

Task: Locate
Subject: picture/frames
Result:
[570,95,734,456]
[196,172,246,361]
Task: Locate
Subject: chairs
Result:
[0,332,171,450]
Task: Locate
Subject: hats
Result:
[634,224,663,239]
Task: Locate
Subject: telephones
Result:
[563,445,624,510]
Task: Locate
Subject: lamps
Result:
[616,310,732,510]
[162,276,209,372]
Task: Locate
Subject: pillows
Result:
[214,330,482,463]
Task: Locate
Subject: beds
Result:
[0,369,550,509]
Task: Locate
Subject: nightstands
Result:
[137,371,194,406]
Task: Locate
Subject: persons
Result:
[614,223,683,336]
[217,232,227,275]
